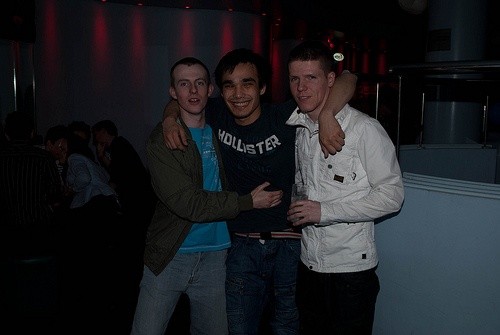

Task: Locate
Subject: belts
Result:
[233,226,302,239]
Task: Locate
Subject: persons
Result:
[129,57,284,334]
[0,110,149,242]
[162,48,356,335]
[287,41,405,334]
